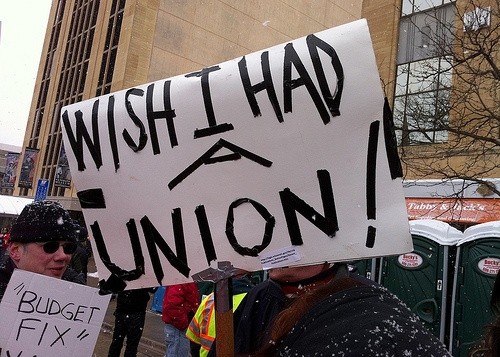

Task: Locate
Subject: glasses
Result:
[42,242,78,255]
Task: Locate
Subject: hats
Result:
[9,201,78,242]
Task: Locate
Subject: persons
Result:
[471,269,500,357]
[207,262,453,357]
[61,226,93,286]
[108,287,159,357]
[0,200,78,304]
[161,273,257,357]
[0,227,11,255]
